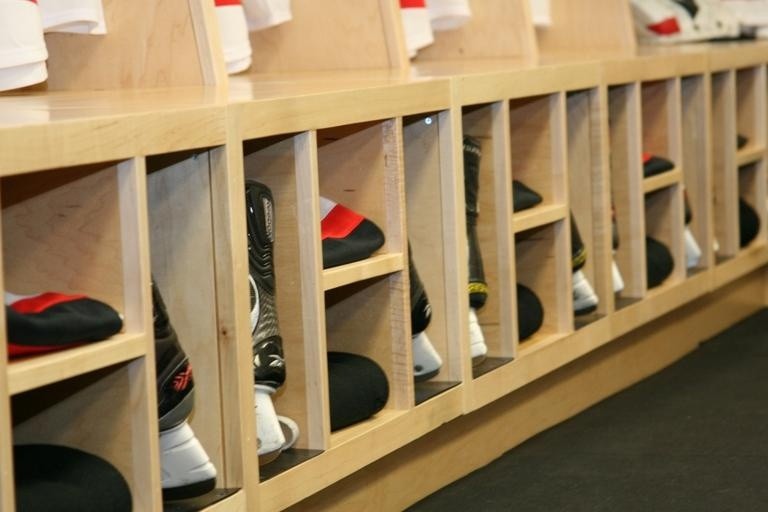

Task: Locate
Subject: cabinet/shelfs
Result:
[2,33,768,511]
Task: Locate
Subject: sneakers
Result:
[407,237,443,341]
[611,206,625,257]
[571,211,600,275]
[684,187,702,227]
[153,280,217,433]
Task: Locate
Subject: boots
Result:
[463,133,488,308]
[245,178,286,393]
